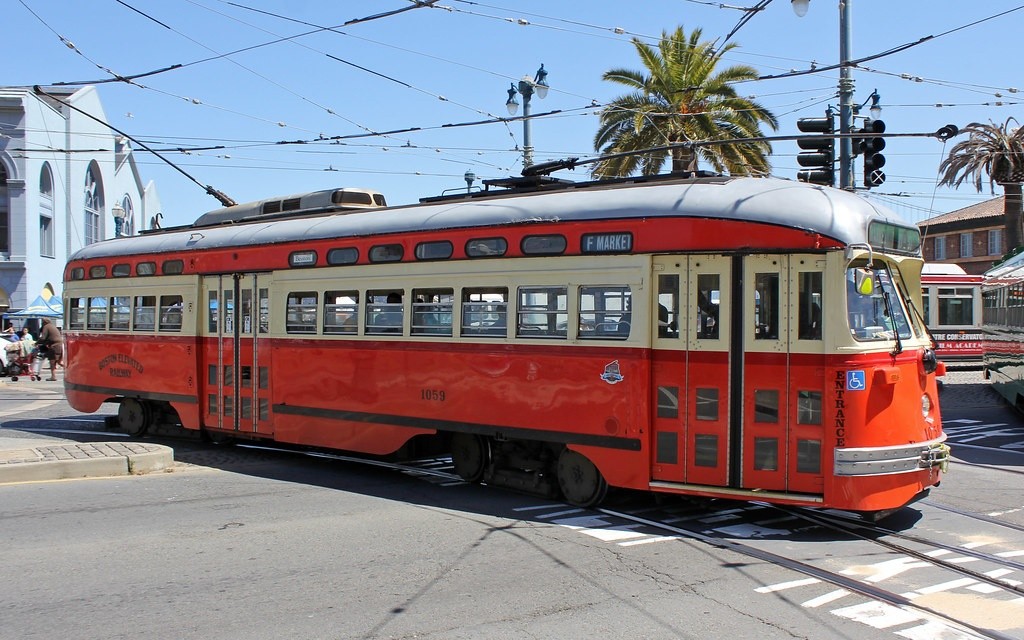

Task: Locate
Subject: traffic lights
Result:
[796,109,837,187]
[852,128,873,155]
[863,117,888,188]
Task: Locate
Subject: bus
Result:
[62,153,952,510]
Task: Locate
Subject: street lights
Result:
[834,86,883,193]
[506,63,553,165]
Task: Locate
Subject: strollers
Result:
[5,343,43,381]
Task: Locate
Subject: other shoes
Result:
[46,377,57,381]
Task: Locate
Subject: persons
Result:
[340,279,889,340]
[2,317,64,381]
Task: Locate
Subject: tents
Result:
[1,286,142,337]
